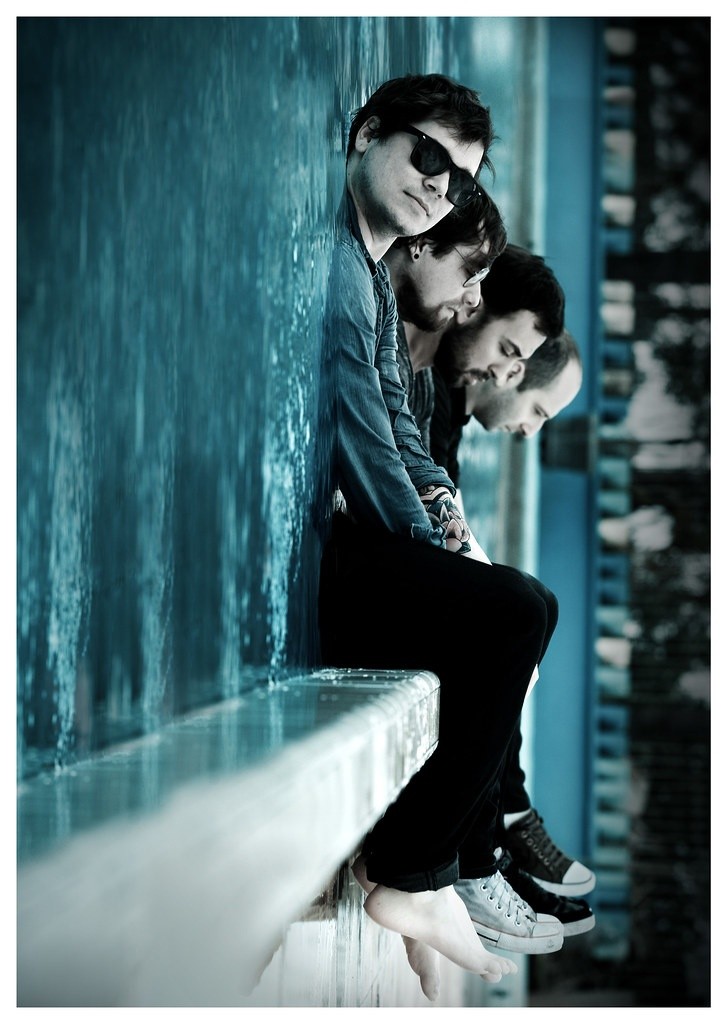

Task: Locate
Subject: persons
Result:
[317,72,560,1001]
[343,73,597,954]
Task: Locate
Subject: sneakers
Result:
[493,850,597,937]
[453,870,564,954]
[503,808,599,898]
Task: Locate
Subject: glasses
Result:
[451,243,490,288]
[399,123,483,209]
